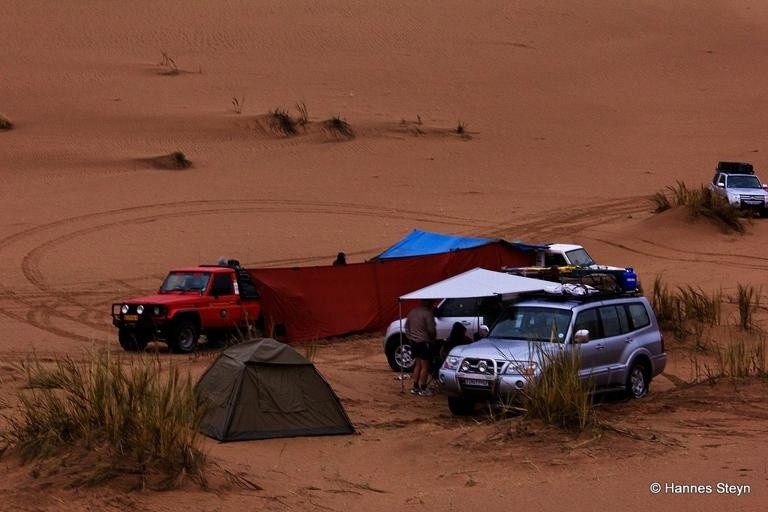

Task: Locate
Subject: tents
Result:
[186,335,356,447]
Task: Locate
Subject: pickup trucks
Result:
[536,243,626,292]
[709,172,768,216]
[112,263,262,354]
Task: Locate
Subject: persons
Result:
[403,298,438,397]
[332,251,347,264]
[439,320,471,364]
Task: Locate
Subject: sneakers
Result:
[409,384,435,396]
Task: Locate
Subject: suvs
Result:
[383,292,667,416]
